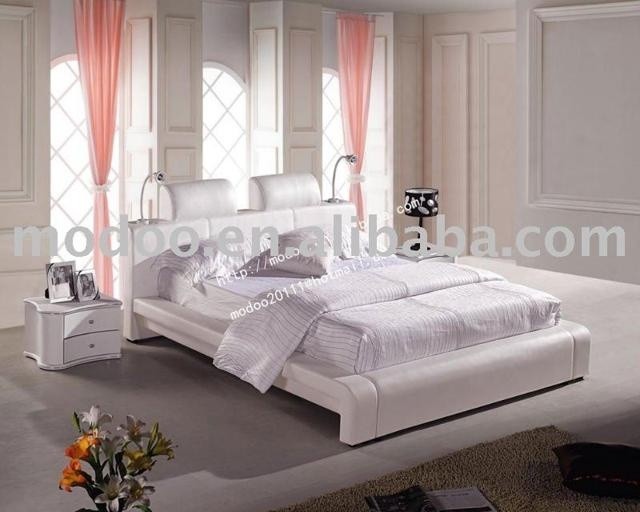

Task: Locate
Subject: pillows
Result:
[153,231,275,303]
[261,221,389,276]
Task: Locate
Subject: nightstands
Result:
[395,245,454,264]
[21,294,124,370]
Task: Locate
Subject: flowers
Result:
[57,402,176,511]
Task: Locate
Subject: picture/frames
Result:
[45,259,76,304]
[73,269,101,302]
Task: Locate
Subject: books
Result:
[364,484,496,511]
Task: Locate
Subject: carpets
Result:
[265,420,640,512]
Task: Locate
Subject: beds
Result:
[121,167,594,447]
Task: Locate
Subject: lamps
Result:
[136,169,170,226]
[401,181,440,254]
[328,154,358,203]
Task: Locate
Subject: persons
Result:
[55,267,69,285]
[79,274,94,297]
